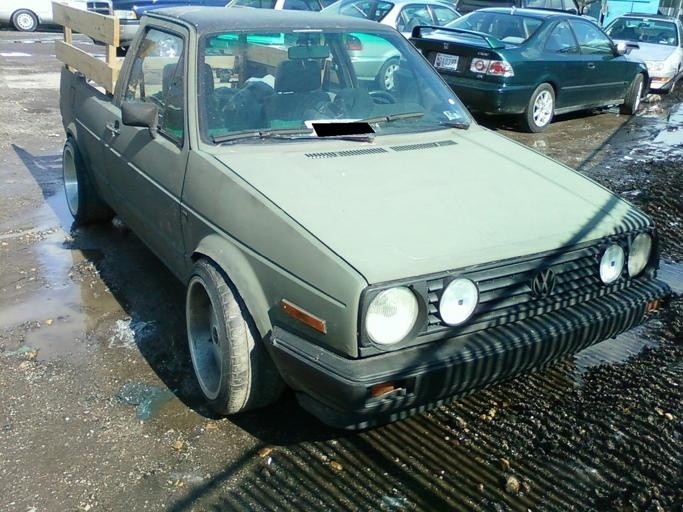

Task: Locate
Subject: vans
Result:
[451,1,579,40]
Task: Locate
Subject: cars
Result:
[588,13,683,94]
[408,7,650,134]
[85,0,231,56]
[201,0,337,57]
[295,1,484,94]
[0,0,87,32]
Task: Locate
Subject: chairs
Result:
[160,61,218,131]
[264,60,331,125]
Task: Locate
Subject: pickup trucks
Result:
[52,2,673,432]
